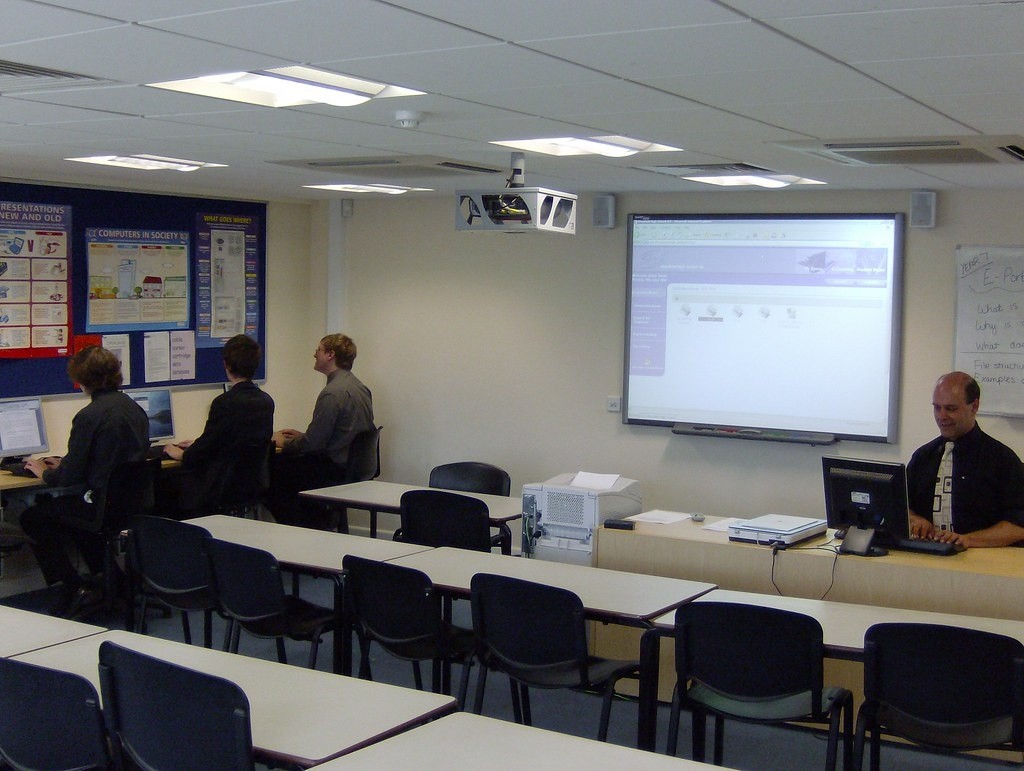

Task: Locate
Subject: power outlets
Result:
[607,395,621,412]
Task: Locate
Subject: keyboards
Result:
[10,466,40,478]
[872,539,967,556]
[155,443,187,461]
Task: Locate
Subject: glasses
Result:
[316,349,333,355]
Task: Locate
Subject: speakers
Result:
[593,195,617,228]
[910,191,936,229]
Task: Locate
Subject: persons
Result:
[263,333,373,528]
[163,334,274,520]
[20,346,149,619]
[904,371,1024,549]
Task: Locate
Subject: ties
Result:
[933,442,953,534]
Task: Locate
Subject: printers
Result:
[524,471,641,567]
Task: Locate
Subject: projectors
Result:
[468,198,562,220]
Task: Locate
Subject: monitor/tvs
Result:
[117,389,176,446]
[822,454,911,558]
[0,396,50,470]
[223,382,260,393]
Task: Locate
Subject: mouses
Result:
[834,529,848,540]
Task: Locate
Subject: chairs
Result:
[60,454,169,628]
[298,425,383,531]
[665,602,853,771]
[339,555,476,697]
[204,535,335,669]
[0,656,115,771]
[392,489,492,553]
[471,574,641,743]
[98,640,254,771]
[127,512,233,649]
[854,622,1024,771]
[194,440,277,520]
[428,462,512,556]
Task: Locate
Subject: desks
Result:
[642,589,1024,771]
[336,546,717,757]
[0,604,108,660]
[299,480,522,558]
[121,514,435,676]
[303,710,736,771]
[7,628,460,771]
[0,447,283,491]
